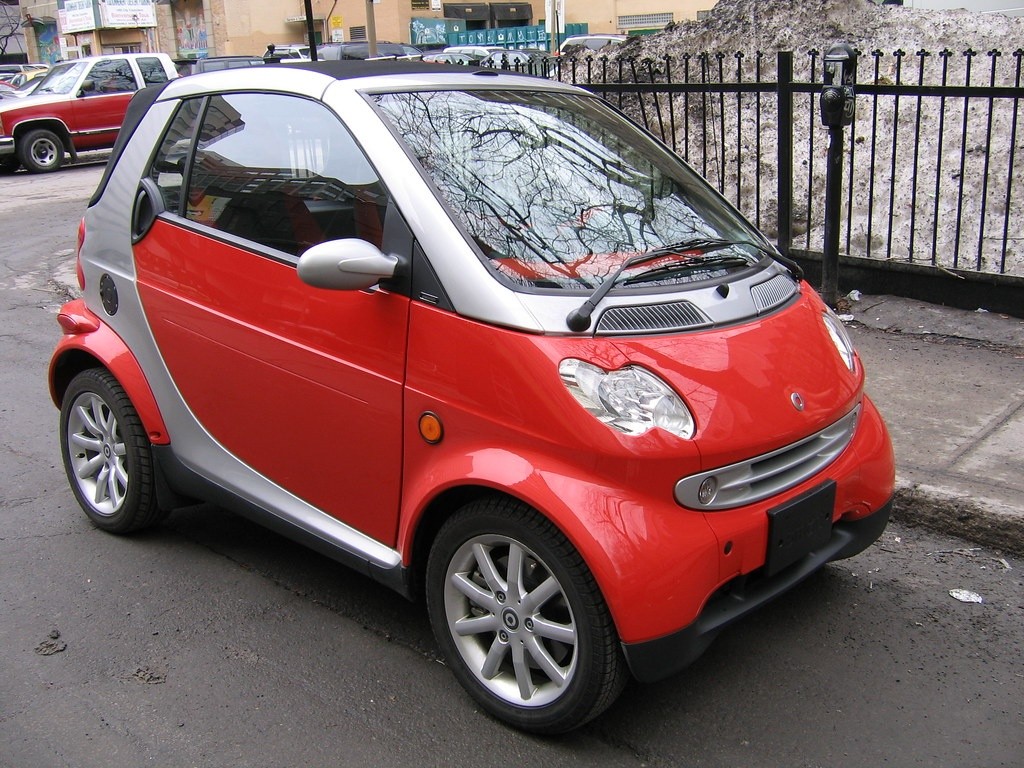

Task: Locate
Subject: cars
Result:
[0,31,629,103]
[47,54,896,737]
[1,53,182,174]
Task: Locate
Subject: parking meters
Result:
[817,41,857,308]
